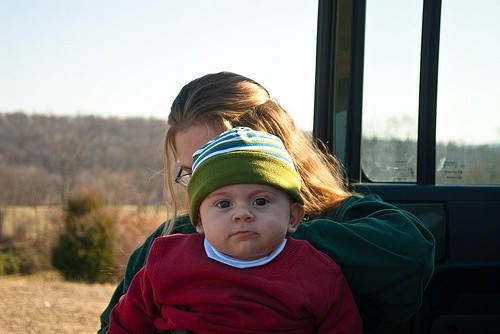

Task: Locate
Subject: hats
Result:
[186,126,305,227]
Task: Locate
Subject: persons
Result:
[104,127,363,334]
[94,71,439,334]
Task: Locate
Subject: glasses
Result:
[175,168,192,189]
